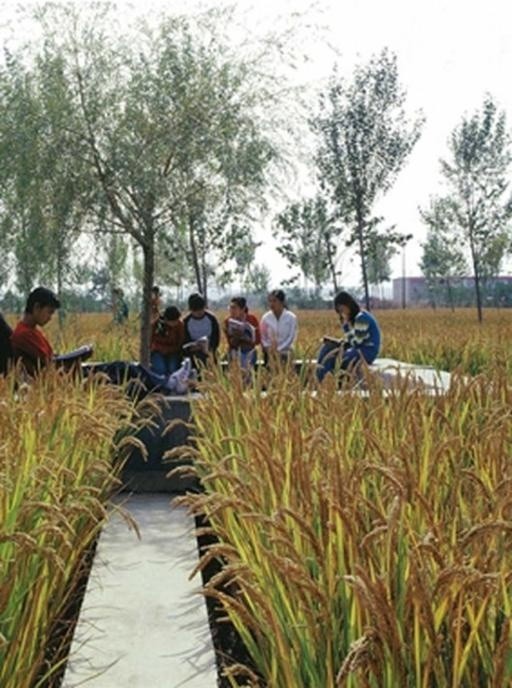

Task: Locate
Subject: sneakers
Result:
[170,358,197,395]
